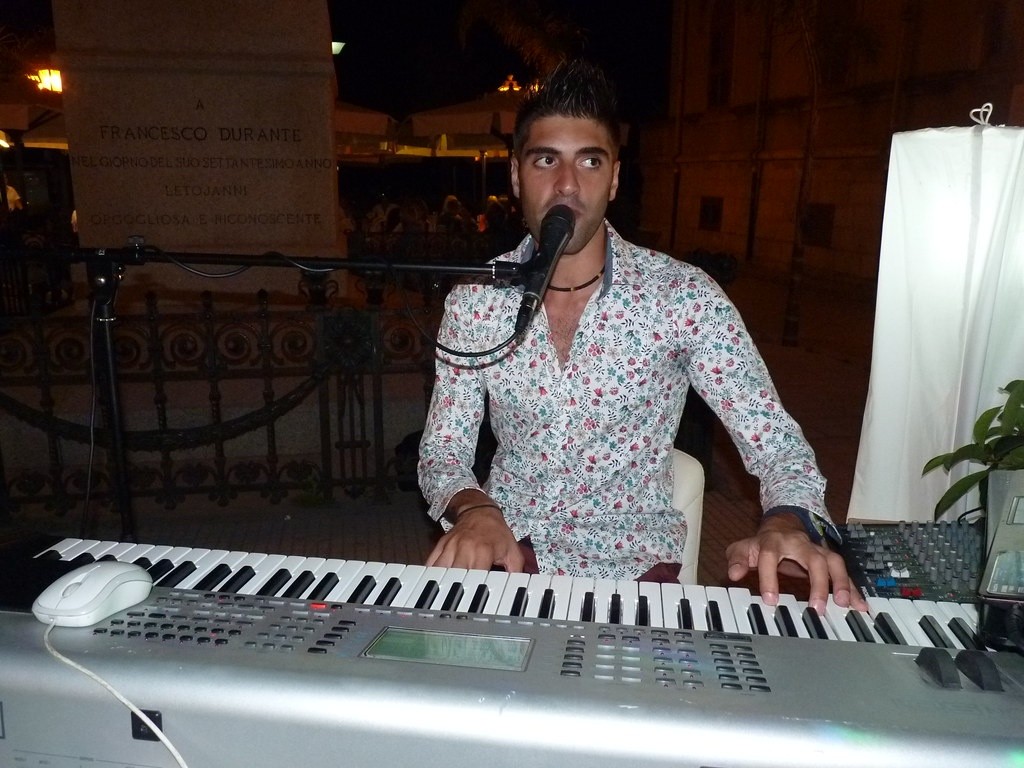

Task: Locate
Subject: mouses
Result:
[31,561,153,627]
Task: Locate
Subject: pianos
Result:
[0,525,1024,766]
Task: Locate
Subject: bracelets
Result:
[456,504,503,521]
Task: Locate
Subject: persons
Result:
[416,56,868,617]
[337,188,523,306]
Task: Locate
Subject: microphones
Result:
[513,204,575,335]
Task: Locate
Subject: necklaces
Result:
[547,264,607,293]
